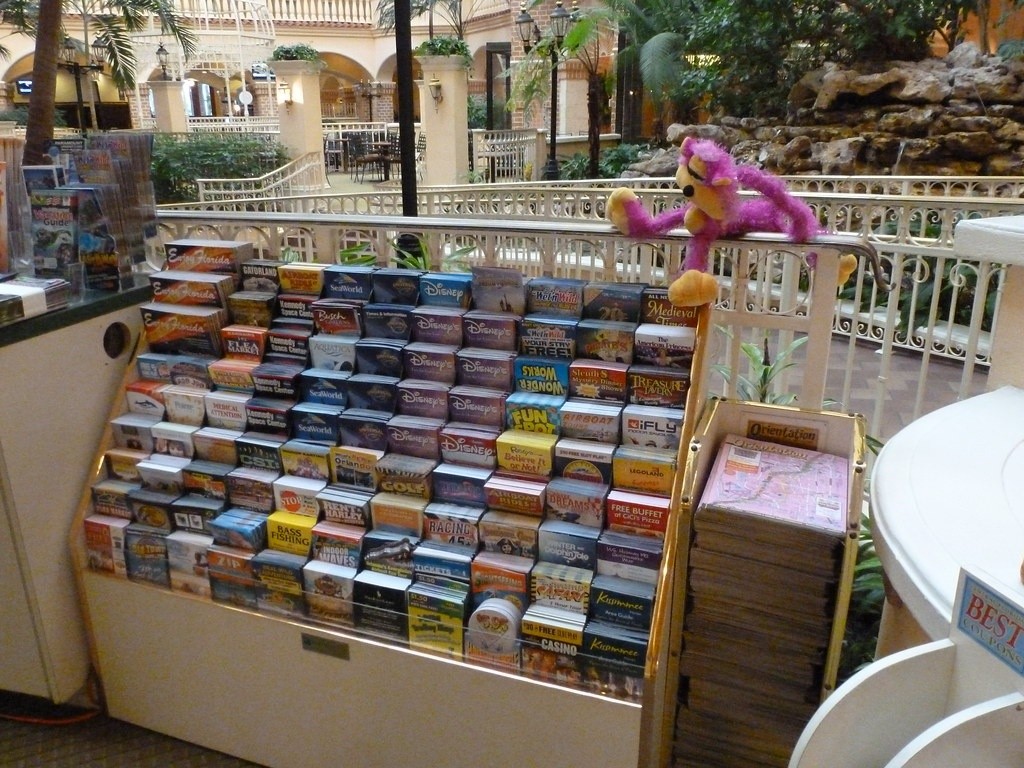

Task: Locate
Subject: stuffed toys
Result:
[606,136,858,306]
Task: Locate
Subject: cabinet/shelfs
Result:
[0,274,157,705]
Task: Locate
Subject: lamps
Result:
[154,38,170,81]
[278,77,293,115]
[429,73,443,113]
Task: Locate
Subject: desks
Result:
[361,142,392,182]
[332,139,349,173]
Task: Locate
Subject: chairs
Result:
[321,130,425,184]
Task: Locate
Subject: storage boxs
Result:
[83,240,705,703]
